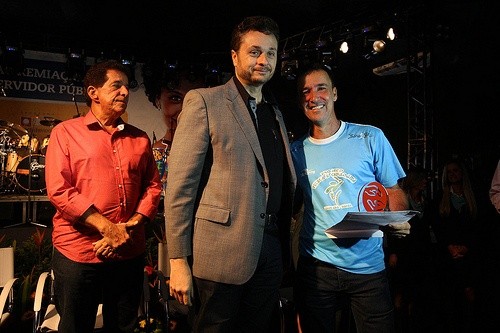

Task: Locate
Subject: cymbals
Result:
[40,118,62,127]
[0,119,28,134]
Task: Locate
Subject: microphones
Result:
[72,92,75,101]
[1,88,7,96]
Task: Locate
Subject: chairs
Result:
[33,269,106,333]
[147,242,189,333]
[0,243,20,329]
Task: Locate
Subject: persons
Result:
[142,62,208,153]
[286,65,411,333]
[432,161,482,301]
[488,159,500,217]
[165,15,295,333]
[386,165,427,315]
[43,60,161,333]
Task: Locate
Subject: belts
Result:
[265,215,278,224]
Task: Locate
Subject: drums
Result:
[41,138,50,155]
[15,154,47,192]
[18,135,40,157]
[0,150,22,178]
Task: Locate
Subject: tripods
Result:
[3,119,47,229]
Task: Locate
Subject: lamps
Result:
[65,48,227,88]
[0,39,27,76]
[279,10,398,81]
